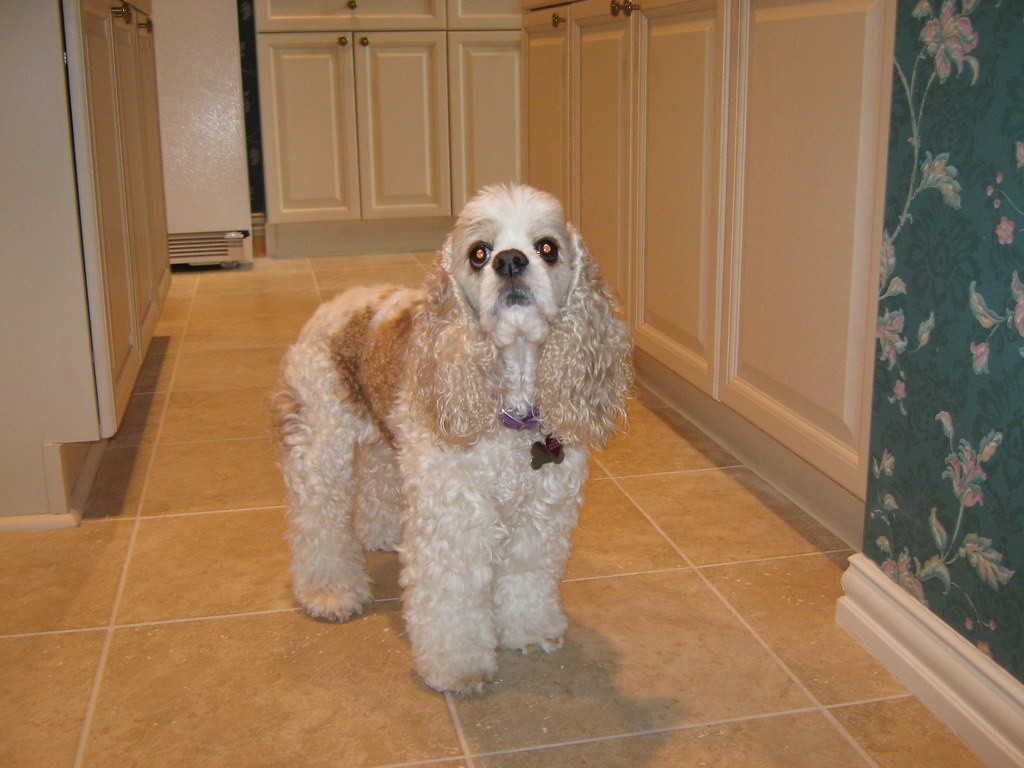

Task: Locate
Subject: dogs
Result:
[272,181,635,695]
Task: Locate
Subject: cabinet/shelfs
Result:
[521,0,898,504]
[1,0,172,447]
[252,0,522,226]
